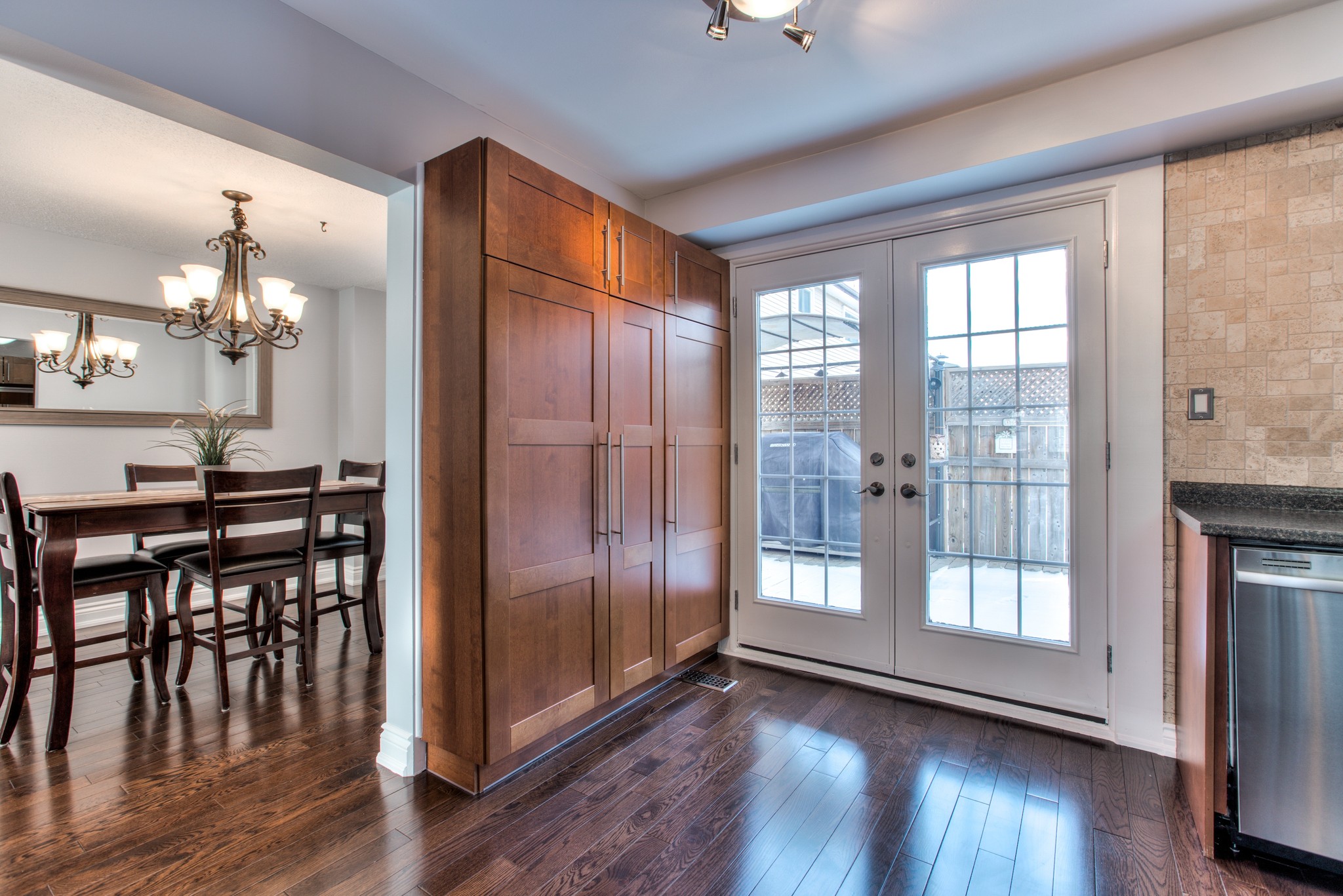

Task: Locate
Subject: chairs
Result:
[0,460,386,747]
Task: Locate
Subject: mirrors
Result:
[0,285,273,428]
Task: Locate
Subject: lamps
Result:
[30,313,140,389]
[702,0,816,53]
[158,190,308,365]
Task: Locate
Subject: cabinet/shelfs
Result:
[424,137,730,799]
[0,355,34,384]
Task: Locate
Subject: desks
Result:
[15,480,387,753]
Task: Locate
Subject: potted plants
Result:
[142,399,274,490]
